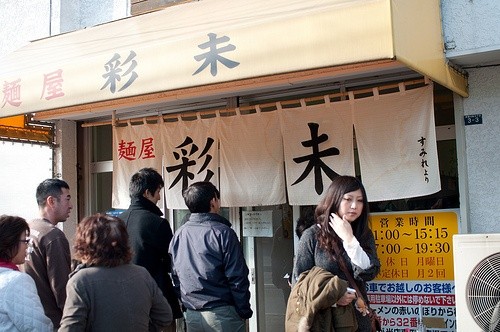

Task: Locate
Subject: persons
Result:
[0,215,54,332]
[292,175,381,332]
[57,214,173,332]
[116,168,184,332]
[168,182,253,332]
[17,179,71,332]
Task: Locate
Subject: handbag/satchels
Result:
[354,297,380,332]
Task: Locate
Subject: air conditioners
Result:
[453,233,500,332]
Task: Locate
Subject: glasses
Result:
[19,239,30,246]
